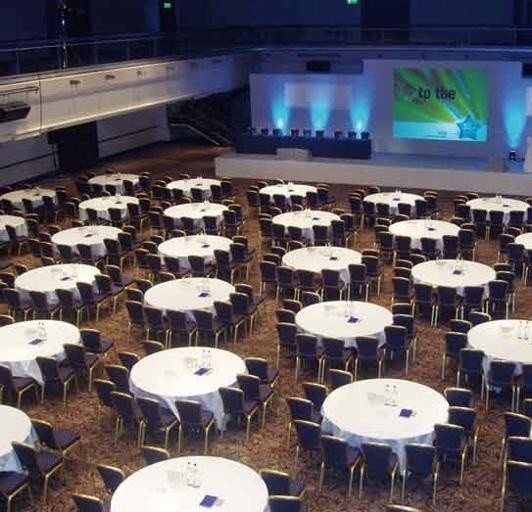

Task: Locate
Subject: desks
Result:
[229,131,372,160]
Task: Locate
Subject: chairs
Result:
[0,167,531,512]
[247,127,370,140]
